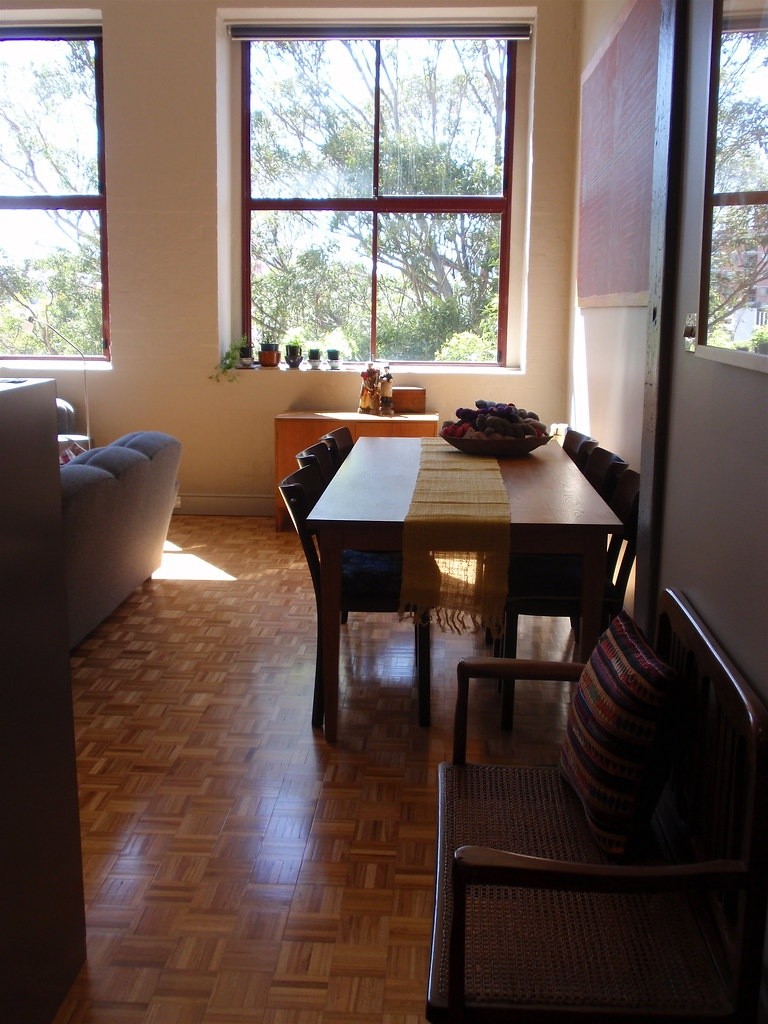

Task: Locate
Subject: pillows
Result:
[69,441,86,457]
[59,450,74,466]
[559,610,685,864]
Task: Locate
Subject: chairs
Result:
[277,425,630,729]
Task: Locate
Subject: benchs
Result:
[424,587,768,1024]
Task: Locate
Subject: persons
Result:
[358,363,393,410]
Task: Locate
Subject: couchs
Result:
[56,397,182,652]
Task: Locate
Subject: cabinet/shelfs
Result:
[274,411,439,533]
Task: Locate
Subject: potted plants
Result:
[285,338,302,356]
[209,332,255,383]
[259,336,279,351]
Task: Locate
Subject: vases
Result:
[240,358,254,366]
[327,360,342,369]
[309,348,320,360]
[285,356,303,368]
[327,349,339,360]
[258,351,282,367]
[308,360,322,368]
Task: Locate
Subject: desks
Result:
[307,436,625,745]
